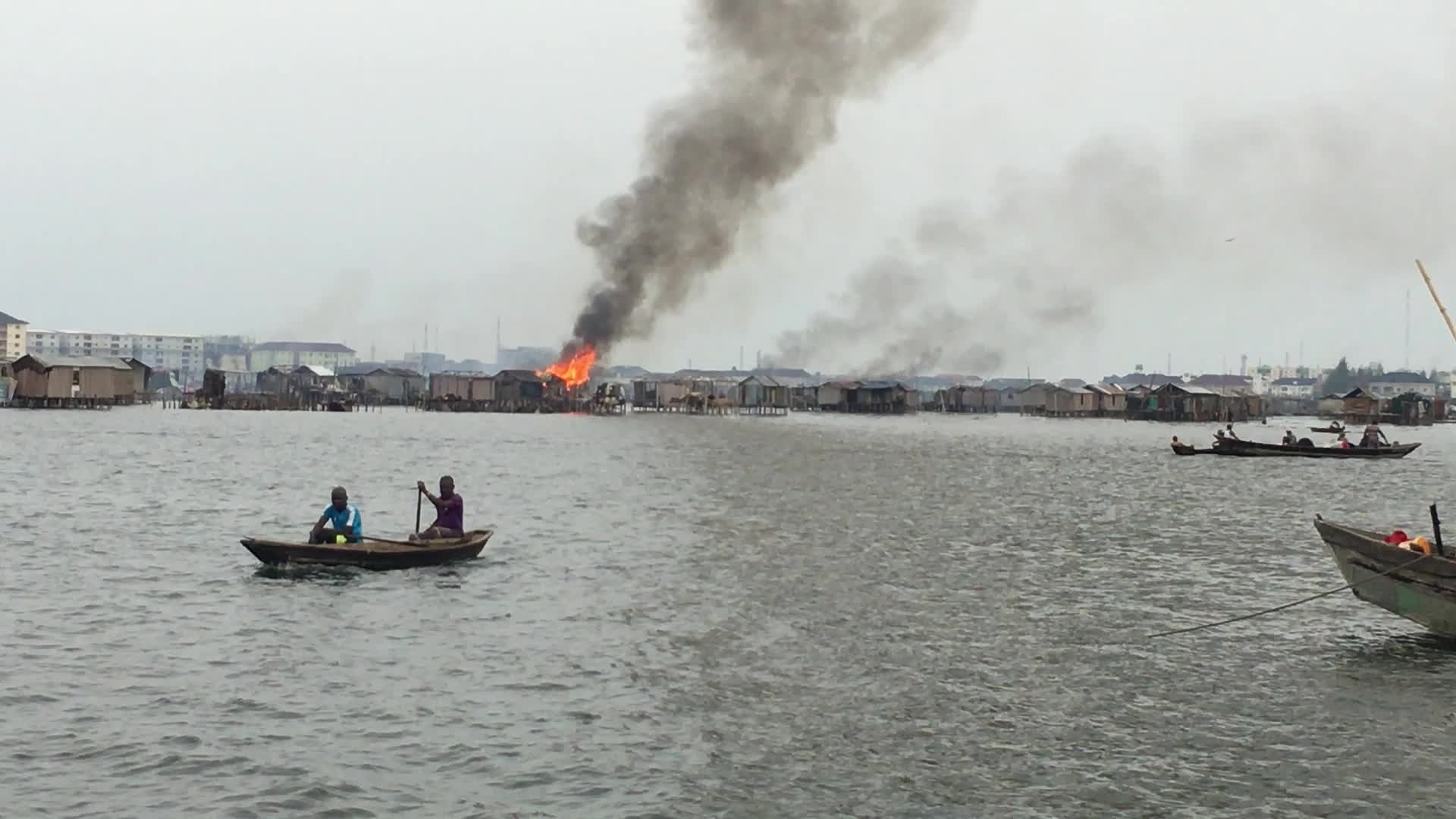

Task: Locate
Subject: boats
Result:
[1310,420,1346,434]
[237,525,498,570]
[1208,430,1421,460]
[1172,443,1220,456]
[1312,514,1456,642]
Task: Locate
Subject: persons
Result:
[1337,431,1354,448]
[1286,429,1297,445]
[1364,420,1385,449]
[308,487,364,544]
[409,475,464,540]
[1171,435,1184,448]
[1223,425,1239,440]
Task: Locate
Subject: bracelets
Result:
[427,491,431,497]
